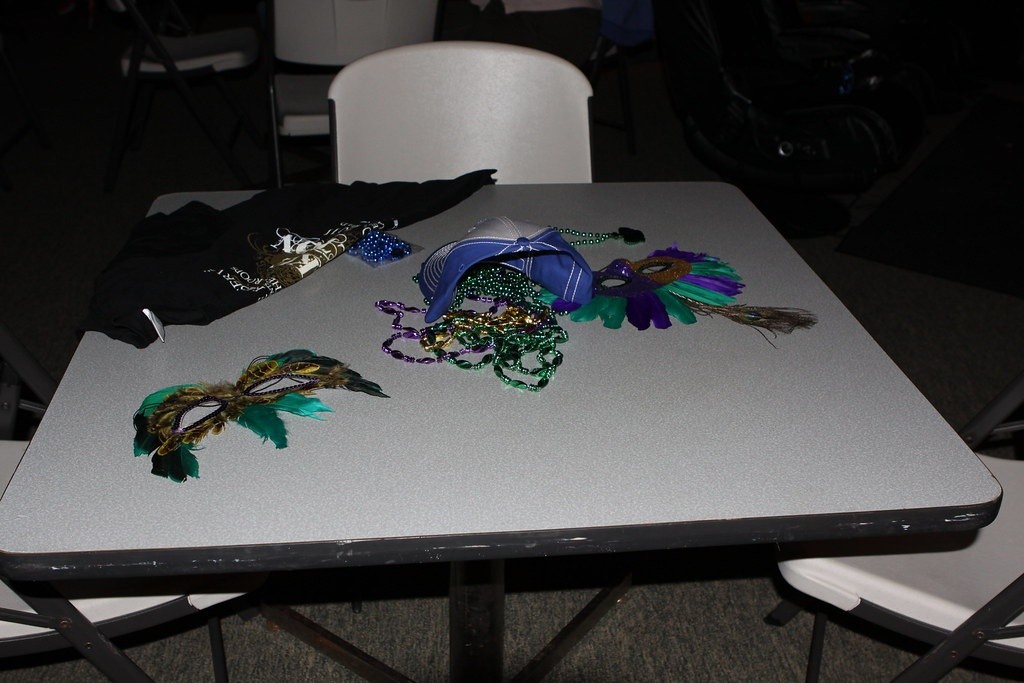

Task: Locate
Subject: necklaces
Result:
[348,235,569,393]
[551,223,650,246]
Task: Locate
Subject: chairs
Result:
[765,374,1024,683]
[1,321,247,682]
[106,0,596,184]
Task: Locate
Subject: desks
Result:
[1,179,1003,683]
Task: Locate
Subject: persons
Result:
[473,0,602,68]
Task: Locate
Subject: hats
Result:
[418,217,592,323]
